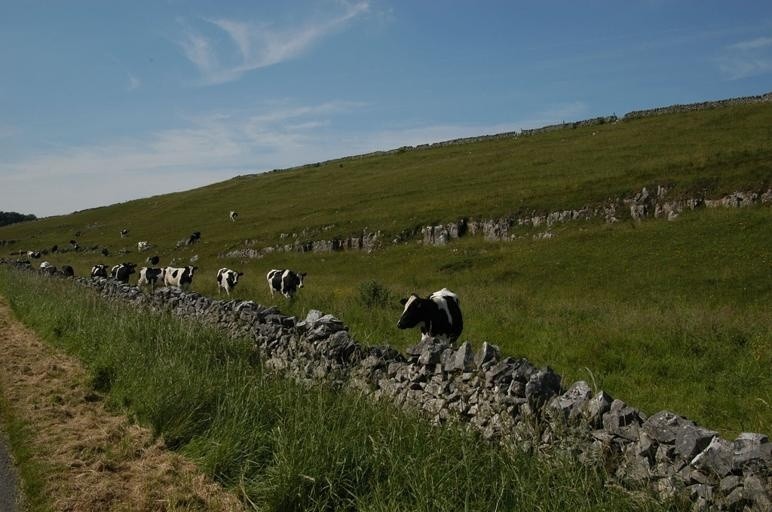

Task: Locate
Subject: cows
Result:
[395,287,464,344]
[185,231,201,247]
[145,255,161,265]
[91,263,109,278]
[137,241,151,250]
[216,268,244,296]
[266,268,307,299]
[111,262,137,282]
[120,228,129,238]
[137,266,165,286]
[229,210,239,222]
[27,250,34,260]
[164,264,198,289]
